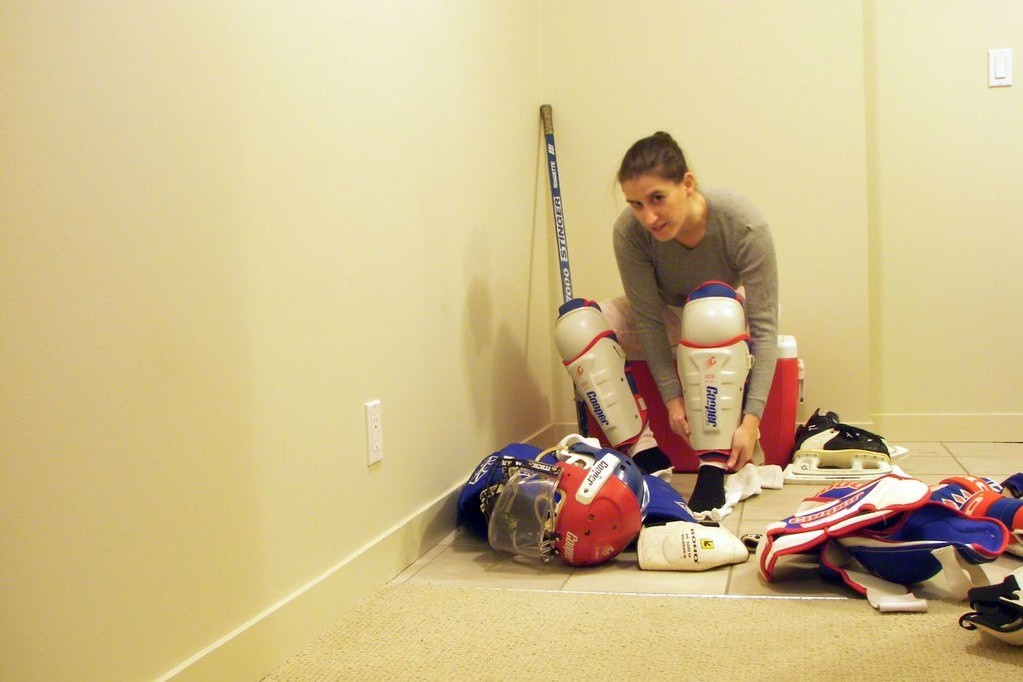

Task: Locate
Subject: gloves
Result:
[930,472,1022,556]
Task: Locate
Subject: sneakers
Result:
[806,409,837,426]
[792,423,888,469]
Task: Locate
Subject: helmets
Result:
[525,442,648,565]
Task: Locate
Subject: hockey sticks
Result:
[537,102,587,439]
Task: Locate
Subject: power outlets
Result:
[365,400,383,466]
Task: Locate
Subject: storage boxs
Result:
[577,335,805,475]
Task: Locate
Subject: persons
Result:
[552,131,779,513]
